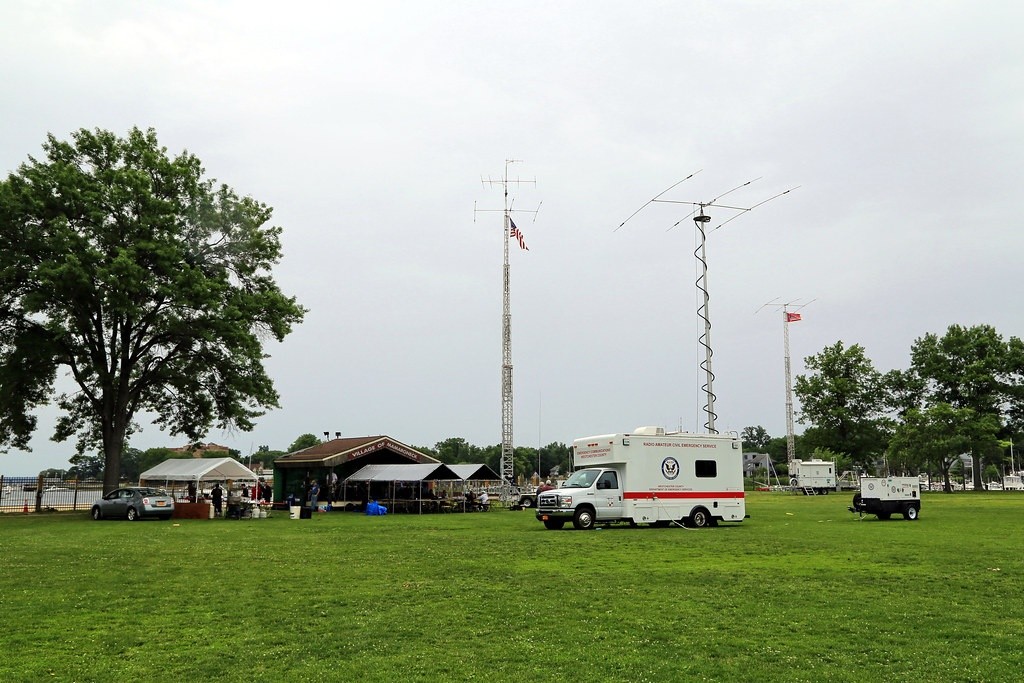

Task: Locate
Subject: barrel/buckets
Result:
[289,505,301,519]
[299,506,313,519]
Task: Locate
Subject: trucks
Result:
[537,426,747,529]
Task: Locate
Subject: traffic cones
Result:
[23,500,29,513]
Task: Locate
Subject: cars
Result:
[90,486,174,520]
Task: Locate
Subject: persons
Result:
[186,472,489,517]
[543,480,553,490]
[536,481,544,495]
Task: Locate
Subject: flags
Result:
[787,313,801,322]
[510,218,529,251]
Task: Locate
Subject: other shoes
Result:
[215,512,222,516]
[310,509,316,512]
